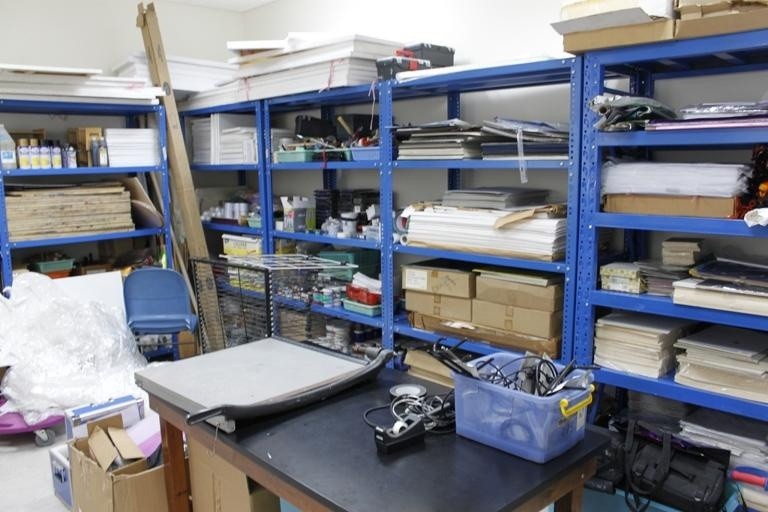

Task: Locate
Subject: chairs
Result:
[123,268,204,362]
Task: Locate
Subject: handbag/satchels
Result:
[611,418,731,511]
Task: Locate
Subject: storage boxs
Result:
[396,257,564,359]
[66,414,192,512]
[546,1,768,56]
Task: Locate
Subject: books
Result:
[395,100,767,512]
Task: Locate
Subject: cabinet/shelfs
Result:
[263,78,382,361]
[387,57,579,379]
[179,97,265,346]
[0,99,175,374]
[574,26,768,510]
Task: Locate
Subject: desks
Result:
[134,333,612,511]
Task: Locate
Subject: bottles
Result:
[326,324,350,354]
[15,134,108,169]
[216,197,250,219]
[277,285,342,308]
